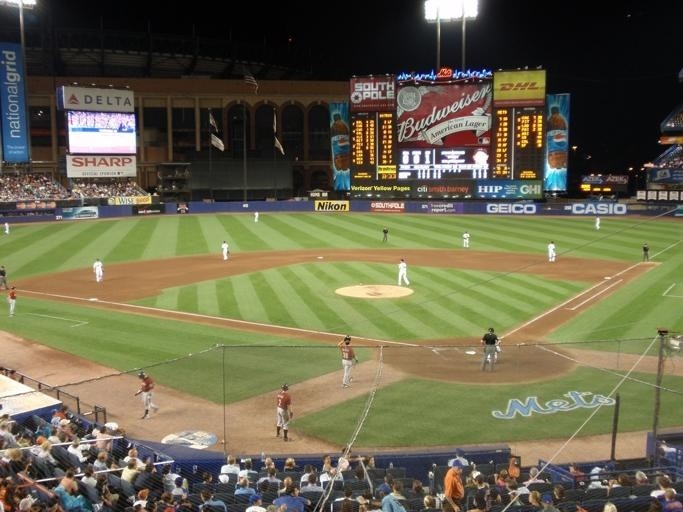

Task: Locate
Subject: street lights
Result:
[424,0,479,75]
[0,1,38,70]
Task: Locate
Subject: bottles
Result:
[545,106,568,170]
[389,462,393,468]
[261,451,265,462]
[330,113,351,171]
[192,465,196,474]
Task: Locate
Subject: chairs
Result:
[0,405,683,512]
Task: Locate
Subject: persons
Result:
[276,384,294,441]
[399,259,409,285]
[481,328,501,372]
[338,336,358,388]
[596,215,601,230]
[221,241,228,261]
[5,222,9,234]
[138,370,159,419]
[1,406,683,512]
[383,227,388,241]
[93,259,104,283]
[9,286,16,315]
[463,232,469,247]
[78,181,140,197]
[548,241,556,262]
[254,211,258,222]
[643,243,649,262]
[0,265,8,289]
[0,173,67,200]
